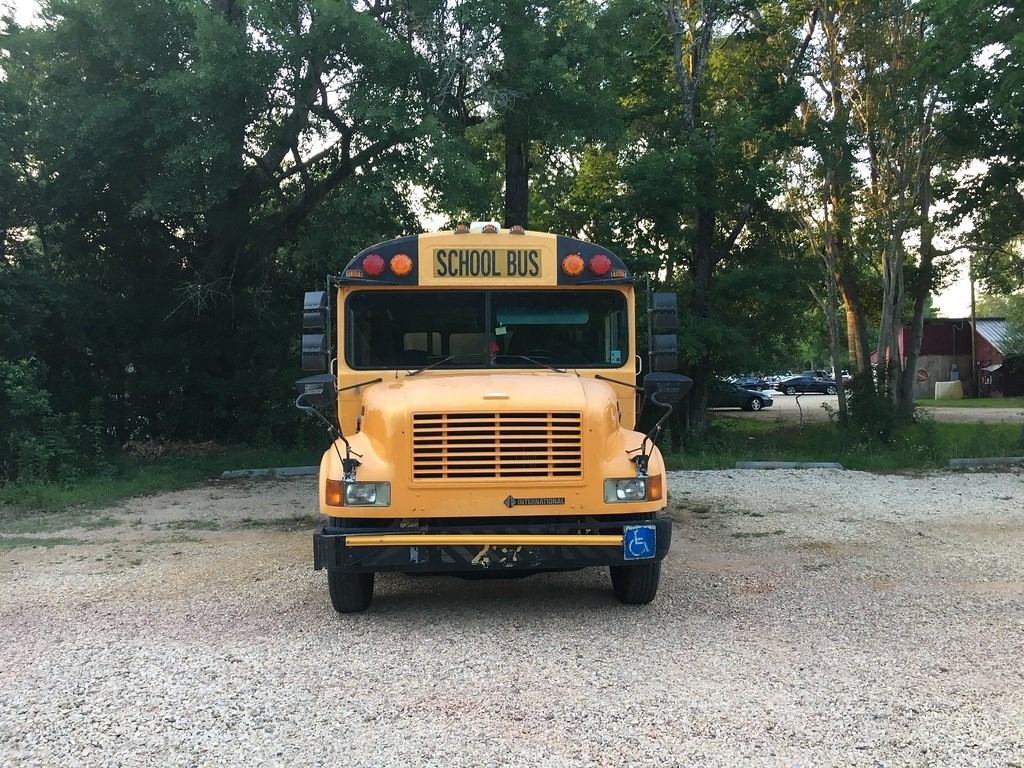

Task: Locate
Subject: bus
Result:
[294,222,693,613]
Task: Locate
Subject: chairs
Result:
[507,324,574,367]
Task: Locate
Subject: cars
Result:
[765,370,837,396]
[707,374,774,411]
